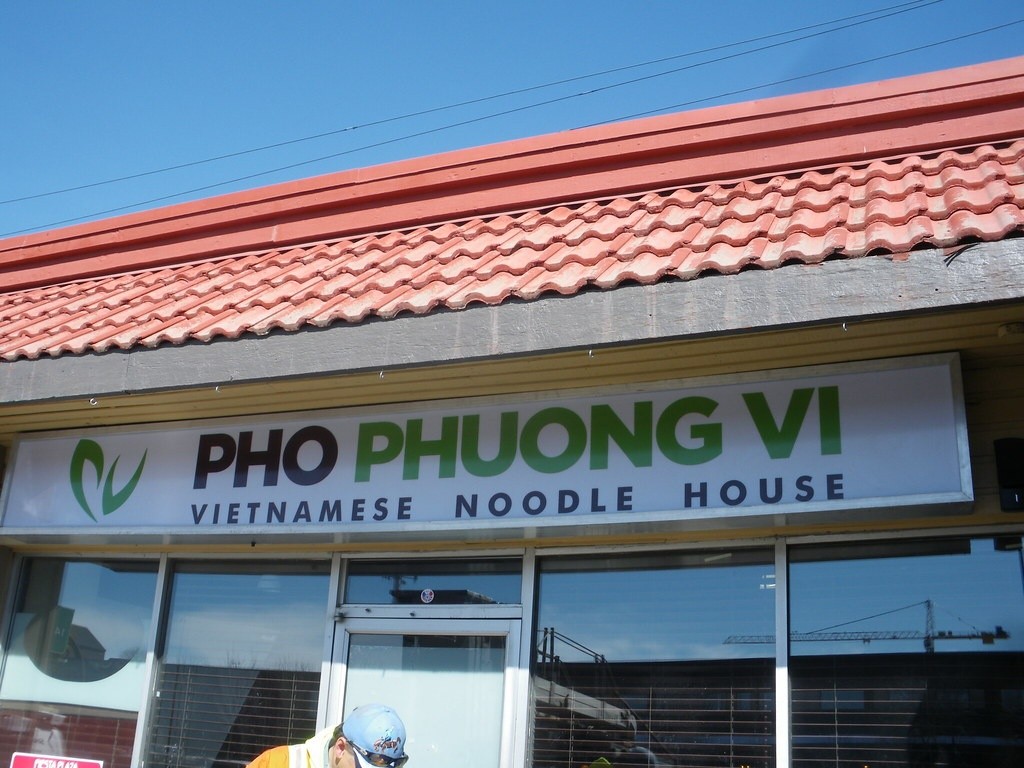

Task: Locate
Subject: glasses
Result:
[344,737,408,768]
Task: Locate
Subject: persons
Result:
[246,704,409,768]
[589,746,656,768]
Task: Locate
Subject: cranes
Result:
[719,597,1012,730]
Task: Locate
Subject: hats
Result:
[342,704,407,768]
